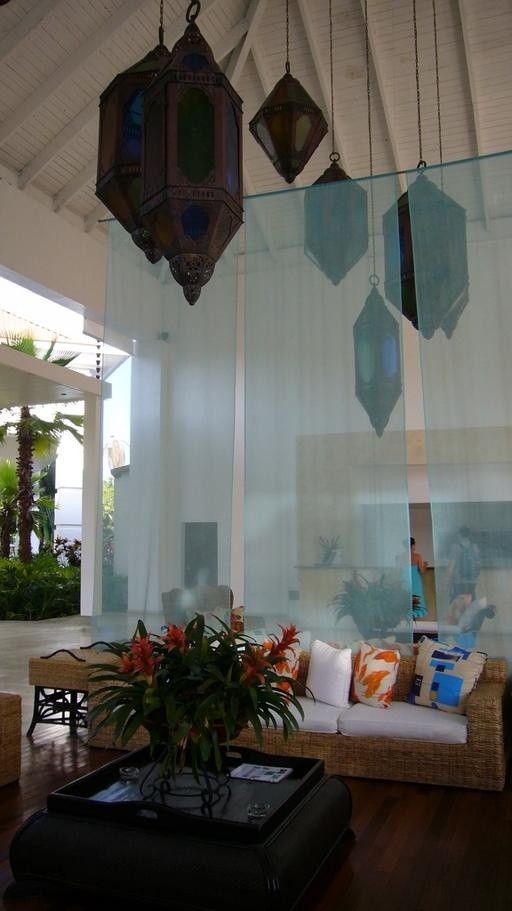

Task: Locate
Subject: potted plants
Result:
[316,534,346,563]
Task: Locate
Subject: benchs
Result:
[161,585,265,630]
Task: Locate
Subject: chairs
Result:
[373,604,498,643]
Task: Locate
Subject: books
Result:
[227,763,293,782]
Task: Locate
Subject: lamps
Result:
[352,285,404,437]
[248,72,328,187]
[93,16,245,308]
[301,162,369,287]
[381,174,466,340]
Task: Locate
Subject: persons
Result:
[393,536,428,620]
[442,526,494,650]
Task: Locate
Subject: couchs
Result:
[90,638,507,793]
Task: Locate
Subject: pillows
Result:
[240,633,488,717]
[446,591,489,634]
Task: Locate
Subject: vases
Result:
[141,714,250,746]
[354,619,396,640]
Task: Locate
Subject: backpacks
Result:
[457,540,481,580]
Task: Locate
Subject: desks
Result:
[10,743,360,911]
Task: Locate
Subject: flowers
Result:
[79,609,322,784]
[326,568,426,631]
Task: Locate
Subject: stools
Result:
[24,637,122,740]
